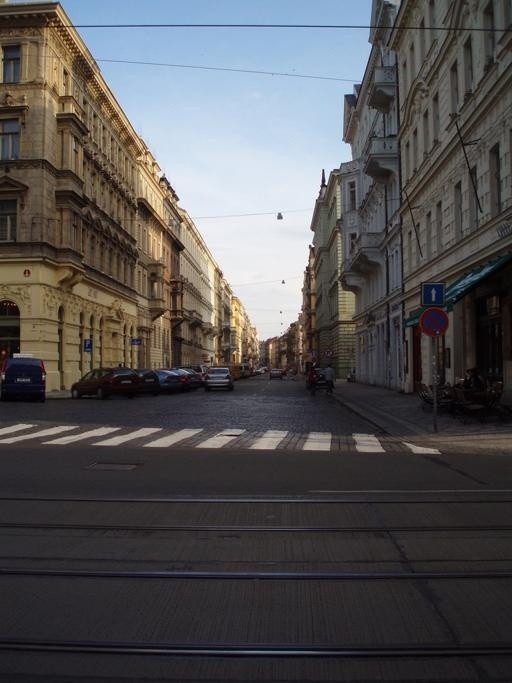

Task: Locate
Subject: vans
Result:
[235,363,251,379]
[2,358,46,401]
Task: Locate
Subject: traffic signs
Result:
[131,339,142,345]
[84,339,93,351]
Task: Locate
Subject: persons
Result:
[307,367,319,397]
[323,364,337,393]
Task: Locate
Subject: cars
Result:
[203,366,234,391]
[304,368,334,389]
[71,366,202,398]
[269,368,284,380]
